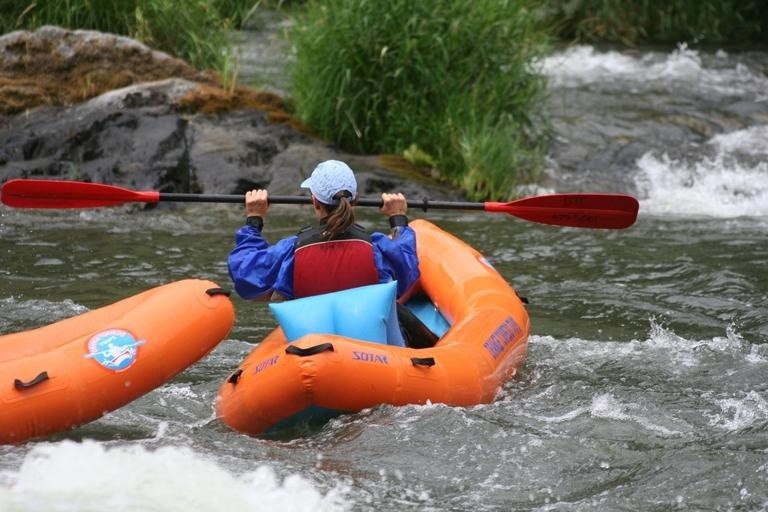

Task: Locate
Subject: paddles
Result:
[0,179,639,229]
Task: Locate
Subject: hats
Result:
[300,159,357,205]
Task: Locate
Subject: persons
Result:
[226,160,420,301]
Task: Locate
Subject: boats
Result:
[214,218,532,436]
[0,278,236,448]
